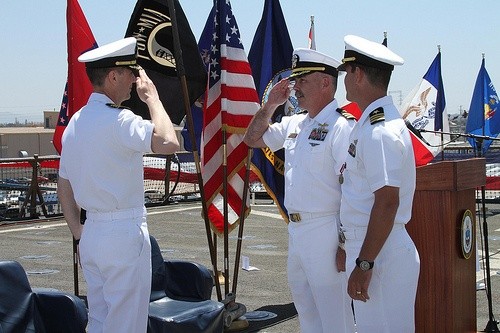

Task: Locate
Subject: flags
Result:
[400,44,451,167]
[342,36,388,121]
[246,0,309,226]
[53,0,100,156]
[123,0,209,125]
[465,52,500,158]
[178,0,270,238]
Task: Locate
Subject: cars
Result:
[0,176,48,184]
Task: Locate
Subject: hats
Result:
[78,37,143,70]
[288,47,346,79]
[337,35,405,72]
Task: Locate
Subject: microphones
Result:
[405,120,431,147]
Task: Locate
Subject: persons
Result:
[243,47,359,333]
[339,33,420,333]
[57,36,180,333]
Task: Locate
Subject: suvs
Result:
[144,190,164,200]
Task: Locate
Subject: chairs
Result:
[0,259,88,333]
[134,235,225,333]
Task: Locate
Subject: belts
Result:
[287,210,339,224]
[339,223,406,241]
[85,207,146,221]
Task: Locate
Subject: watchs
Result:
[355,257,375,272]
